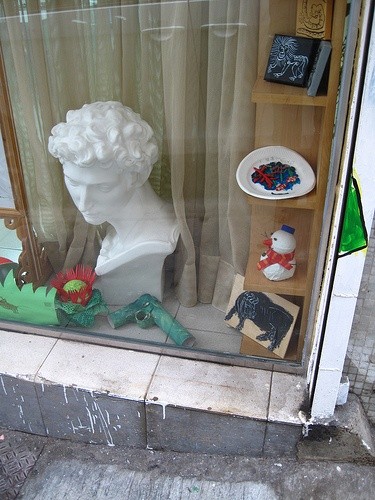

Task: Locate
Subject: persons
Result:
[48,98,182,302]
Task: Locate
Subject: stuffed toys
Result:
[258,223,298,280]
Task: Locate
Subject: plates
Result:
[236,145,316,200]
